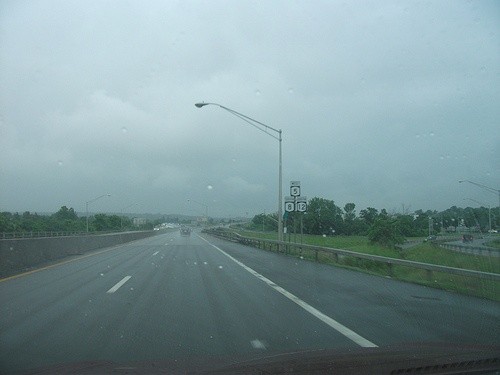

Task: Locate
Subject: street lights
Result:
[429,207,452,235]
[186,198,208,230]
[461,196,492,238]
[86,193,111,234]
[194,101,283,254]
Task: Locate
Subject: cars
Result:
[179,224,191,237]
[423,236,437,243]
[488,229,498,233]
[462,234,474,243]
